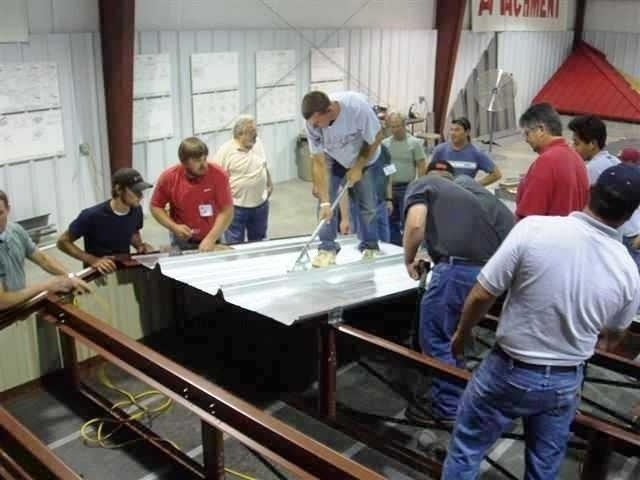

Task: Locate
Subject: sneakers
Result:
[404,404,457,430]
[311,249,336,268]
[359,248,378,264]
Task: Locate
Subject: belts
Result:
[493,341,576,372]
[438,255,486,265]
[188,240,220,244]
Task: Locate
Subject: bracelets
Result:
[317,200,330,213]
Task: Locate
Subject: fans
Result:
[474,69,517,162]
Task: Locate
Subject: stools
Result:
[416,132,441,155]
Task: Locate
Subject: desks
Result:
[406,119,424,136]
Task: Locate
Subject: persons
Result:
[402,159,516,433]
[302,91,383,267]
[617,148,640,312]
[381,113,426,244]
[150,136,234,253]
[440,163,640,479]
[425,117,502,186]
[568,113,640,265]
[517,102,590,216]
[214,116,273,244]
[0,190,92,309]
[56,167,154,278]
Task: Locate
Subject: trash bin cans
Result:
[295,134,314,182]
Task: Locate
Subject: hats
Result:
[454,116,470,142]
[111,168,152,193]
[426,160,455,176]
[615,148,639,162]
[597,163,640,201]
